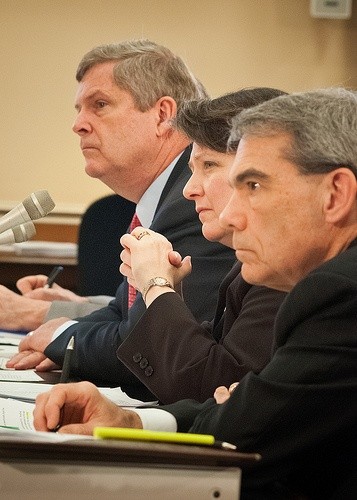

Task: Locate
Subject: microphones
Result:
[0,191,56,233]
[0,222,36,245]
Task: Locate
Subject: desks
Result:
[0,362,260,500]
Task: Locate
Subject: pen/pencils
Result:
[44,266,64,288]
[213,440,237,449]
[52,336,74,432]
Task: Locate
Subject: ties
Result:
[128,213,142,311]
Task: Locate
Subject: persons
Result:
[32,89,357,500]
[1,271,115,337]
[6,39,243,382]
[118,87,293,401]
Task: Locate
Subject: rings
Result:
[136,231,151,240]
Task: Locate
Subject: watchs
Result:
[140,275,175,306]
